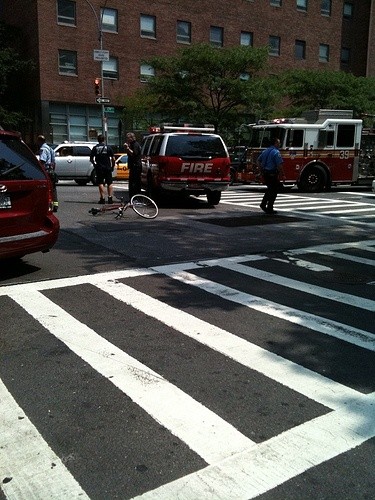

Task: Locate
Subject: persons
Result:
[122,133,143,205]
[35,134,60,212]
[89,134,116,205]
[256,136,286,215]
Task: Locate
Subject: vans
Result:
[234,118,363,193]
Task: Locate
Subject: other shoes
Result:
[98,196,114,204]
[259,203,278,213]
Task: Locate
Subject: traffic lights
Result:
[95,79,100,96]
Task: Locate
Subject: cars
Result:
[0,129,60,262]
[34,142,106,186]
[112,153,130,181]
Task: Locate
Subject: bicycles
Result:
[88,193,159,222]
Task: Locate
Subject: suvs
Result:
[139,123,232,206]
